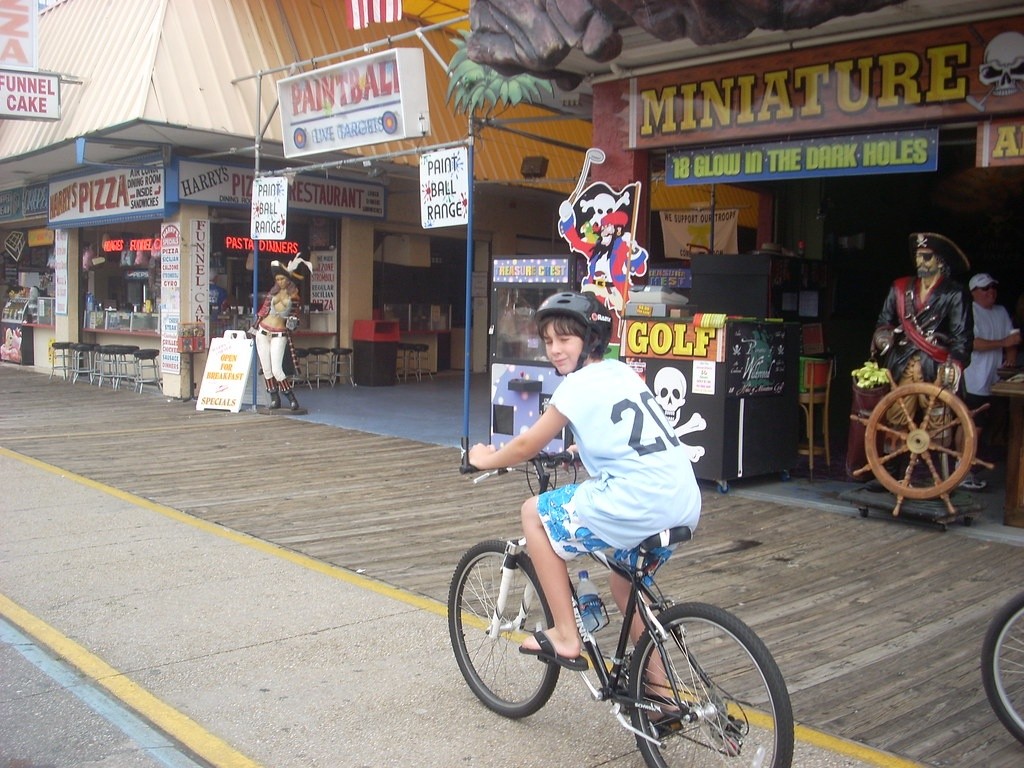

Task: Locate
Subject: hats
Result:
[969,272,998,291]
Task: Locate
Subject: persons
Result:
[874,232,974,493]
[252,252,313,411]
[955,273,1024,489]
[469,291,701,747]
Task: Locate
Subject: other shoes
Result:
[958,478,986,489]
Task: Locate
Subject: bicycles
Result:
[447,435,795,768]
[981,590,1024,746]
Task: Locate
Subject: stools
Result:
[397,343,433,384]
[288,347,355,392]
[799,356,835,482]
[49,342,163,394]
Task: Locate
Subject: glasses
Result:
[978,285,998,292]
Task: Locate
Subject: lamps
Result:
[520,156,550,178]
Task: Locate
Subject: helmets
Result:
[535,291,614,356]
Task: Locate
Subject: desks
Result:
[619,316,798,490]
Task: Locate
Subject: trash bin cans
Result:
[353,319,400,387]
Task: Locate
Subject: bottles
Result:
[575,571,604,632]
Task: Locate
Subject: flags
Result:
[345,0,402,31]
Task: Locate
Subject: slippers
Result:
[651,710,682,726]
[519,631,589,671]
